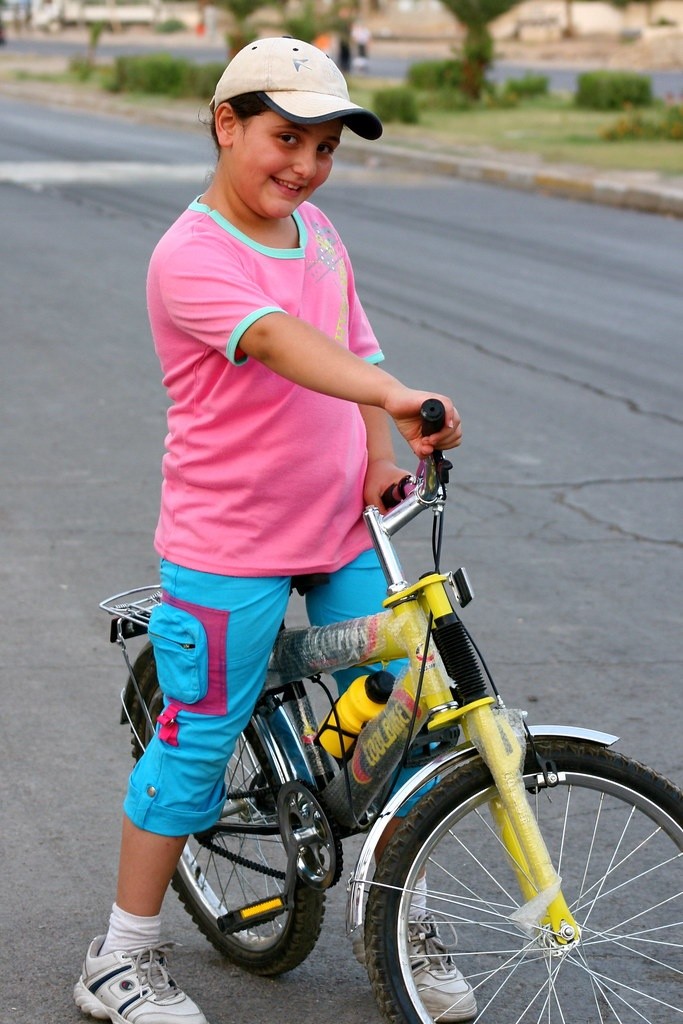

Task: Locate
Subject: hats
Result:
[208,36,384,142]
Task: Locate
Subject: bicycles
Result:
[98,397,683,1023]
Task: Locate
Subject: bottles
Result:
[317,670,396,758]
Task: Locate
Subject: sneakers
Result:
[407,912,479,1020]
[74,934,209,1024]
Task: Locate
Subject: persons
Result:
[73,35,478,1024]
